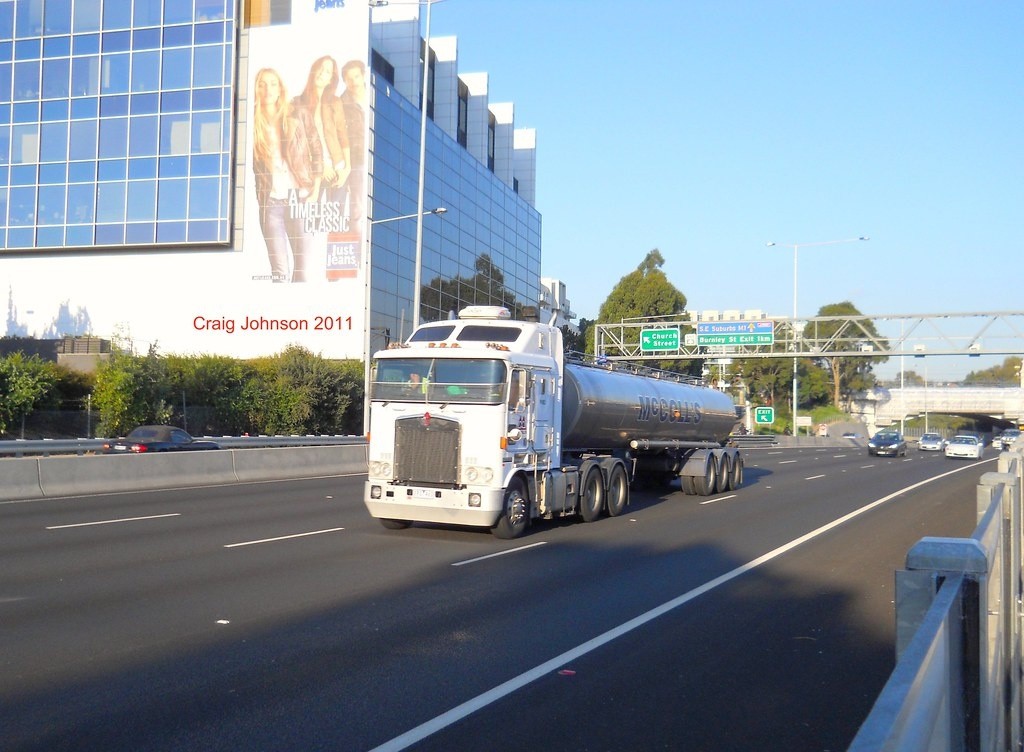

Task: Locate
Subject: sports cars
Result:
[103,425,223,454]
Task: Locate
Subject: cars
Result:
[917,433,948,451]
[991,436,1004,450]
[841,432,864,438]
[868,431,908,457]
[945,435,984,459]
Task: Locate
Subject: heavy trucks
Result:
[363,305,744,540]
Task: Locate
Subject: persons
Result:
[338,59,366,220]
[783,425,792,436]
[252,68,309,285]
[287,55,351,232]
[738,423,749,435]
[401,368,425,394]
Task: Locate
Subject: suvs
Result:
[1000,429,1020,448]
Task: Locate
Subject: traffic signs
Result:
[755,407,774,424]
[697,322,774,346]
[639,328,680,351]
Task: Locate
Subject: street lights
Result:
[361,207,448,435]
[766,236,871,437]
